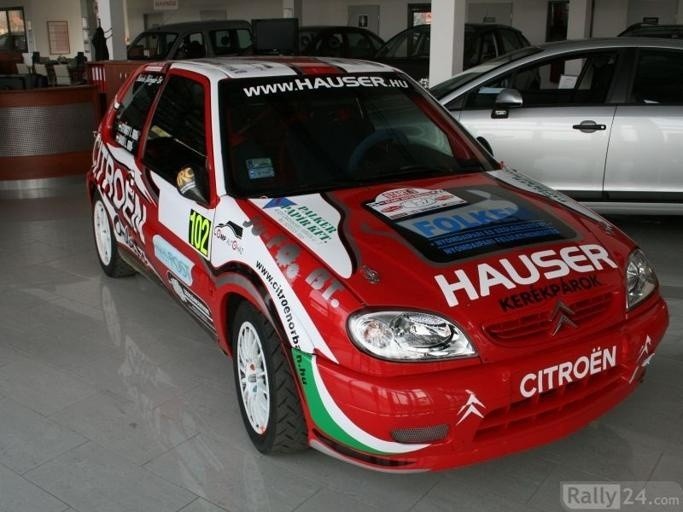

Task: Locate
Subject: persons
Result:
[90,25,109,60]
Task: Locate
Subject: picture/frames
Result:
[45,19,70,56]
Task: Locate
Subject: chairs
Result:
[0,51,88,91]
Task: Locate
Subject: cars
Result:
[84,57,669,474]
[367,23,541,91]
[0,31,26,74]
[377,36,682,219]
[234,26,386,61]
[575,22,682,90]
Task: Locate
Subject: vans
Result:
[126,21,256,61]
